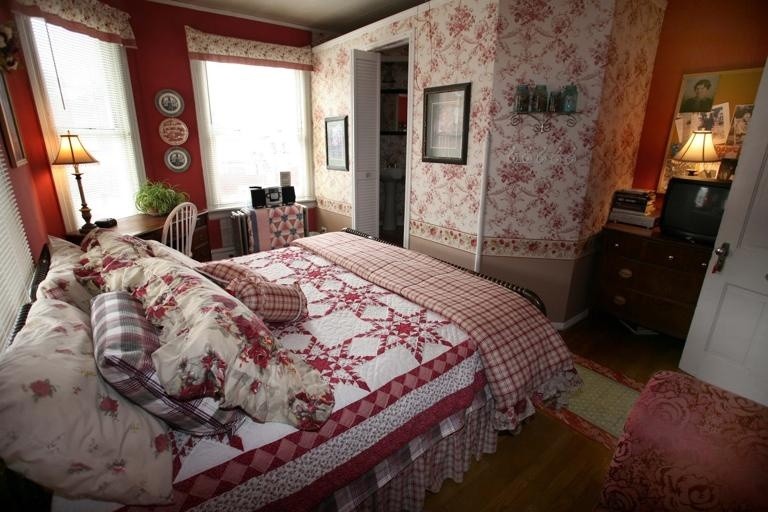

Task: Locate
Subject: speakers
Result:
[251,189,266,209]
[282,186,295,205]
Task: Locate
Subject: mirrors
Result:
[379,88,407,135]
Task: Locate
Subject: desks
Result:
[64,209,213,262]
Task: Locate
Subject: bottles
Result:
[513,83,577,112]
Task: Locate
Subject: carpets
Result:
[530,353,646,454]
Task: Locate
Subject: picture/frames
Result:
[656,66,763,195]
[324,116,349,171]
[421,81,470,165]
[0,69,29,168]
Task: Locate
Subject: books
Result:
[613,189,657,217]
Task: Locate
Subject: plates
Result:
[153,88,191,173]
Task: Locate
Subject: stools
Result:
[592,370,768,512]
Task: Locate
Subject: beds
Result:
[1,228,576,512]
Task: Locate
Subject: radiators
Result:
[231,203,309,256]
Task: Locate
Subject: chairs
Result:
[162,201,198,259]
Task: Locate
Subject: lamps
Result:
[673,130,723,180]
[52,129,100,234]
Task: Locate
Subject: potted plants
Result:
[134,177,190,217]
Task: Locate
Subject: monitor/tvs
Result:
[659,176,732,252]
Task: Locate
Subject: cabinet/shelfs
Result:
[592,226,714,343]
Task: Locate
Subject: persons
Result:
[680,75,714,113]
